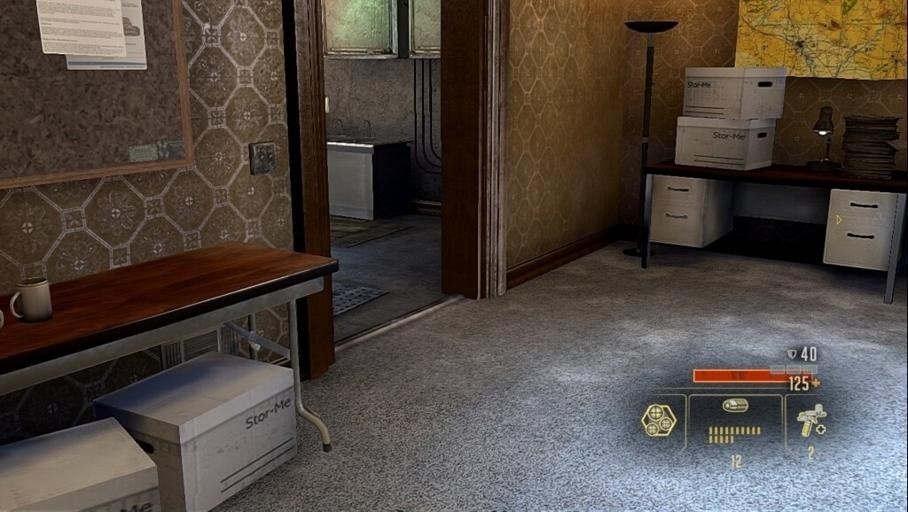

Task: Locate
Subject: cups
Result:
[10,276,53,324]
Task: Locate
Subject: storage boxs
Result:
[93,350,298,512]
[0,418,162,512]
[682,67,787,118]
[675,117,777,170]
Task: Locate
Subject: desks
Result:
[640,158,907,304]
[1,241,339,453]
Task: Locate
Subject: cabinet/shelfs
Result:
[407,0,442,60]
[320,0,407,59]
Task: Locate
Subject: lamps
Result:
[806,106,843,173]
[623,20,681,256]
